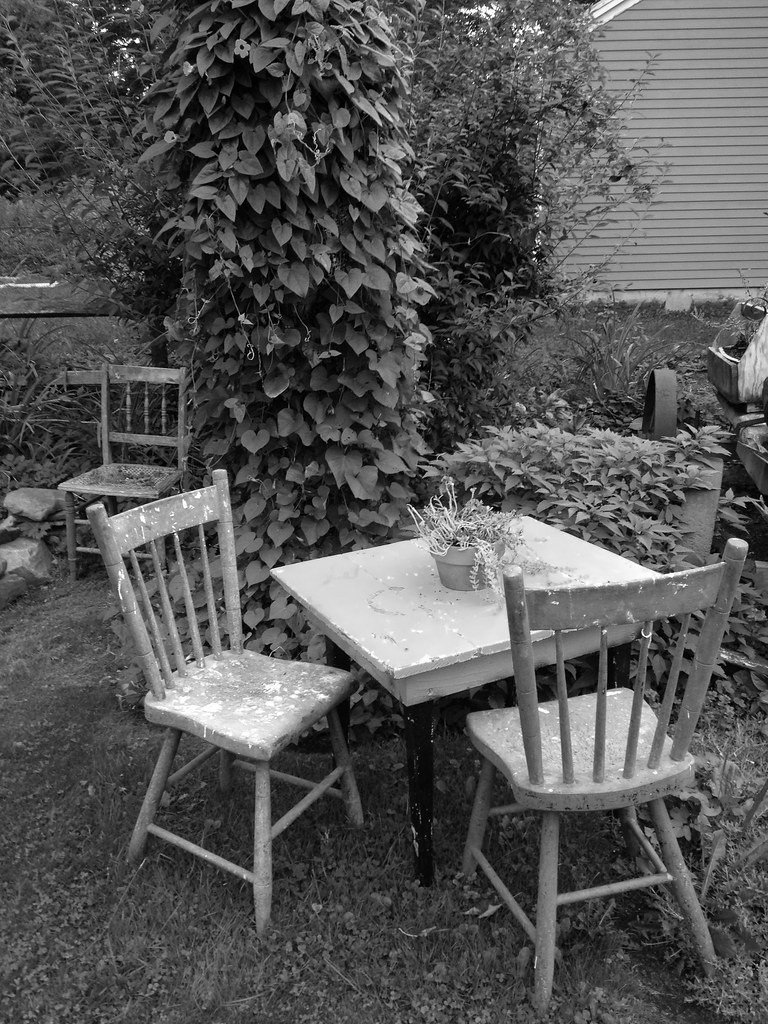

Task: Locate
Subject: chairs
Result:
[455,535,749,1008]
[58,362,190,582]
[86,468,364,931]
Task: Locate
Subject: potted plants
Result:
[407,478,588,612]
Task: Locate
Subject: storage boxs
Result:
[706,300,766,406]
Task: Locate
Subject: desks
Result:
[268,515,668,886]
